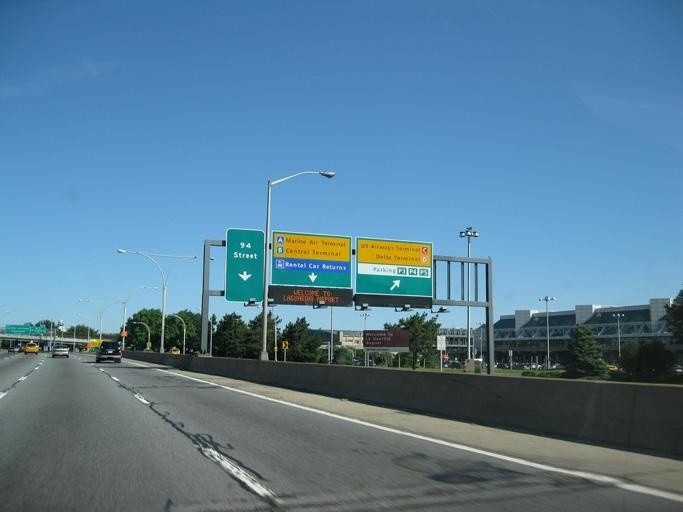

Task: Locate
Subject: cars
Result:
[52,345,70,359]
[8,343,39,355]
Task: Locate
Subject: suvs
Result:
[96,340,122,362]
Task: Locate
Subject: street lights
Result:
[460,226,479,360]
[540,295,554,368]
[78,298,124,339]
[262,172,335,352]
[613,313,624,357]
[361,313,369,328]
[118,248,214,354]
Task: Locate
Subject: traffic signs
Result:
[5,325,46,336]
[225,228,266,301]
[355,237,433,299]
[271,230,351,289]
